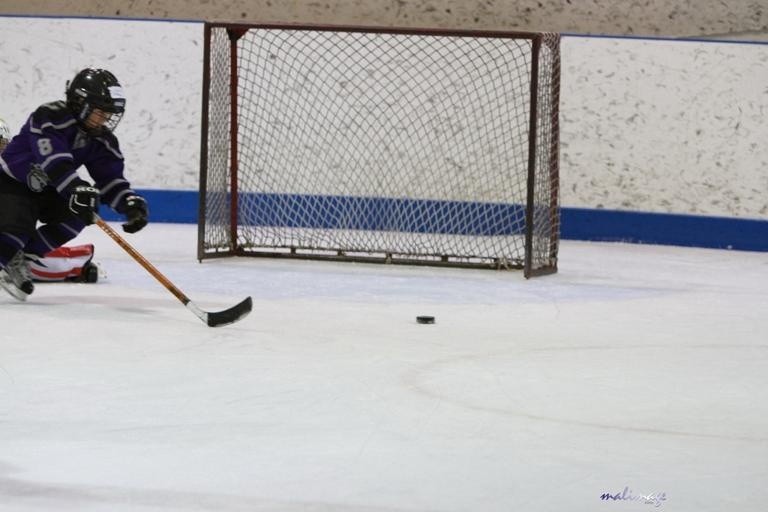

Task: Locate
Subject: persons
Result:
[0,68,150,296]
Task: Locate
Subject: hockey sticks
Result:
[92,213,253,327]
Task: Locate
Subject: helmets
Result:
[64,67,127,139]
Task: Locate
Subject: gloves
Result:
[119,195,149,234]
[68,182,100,224]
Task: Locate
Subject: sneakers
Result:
[3,248,35,294]
